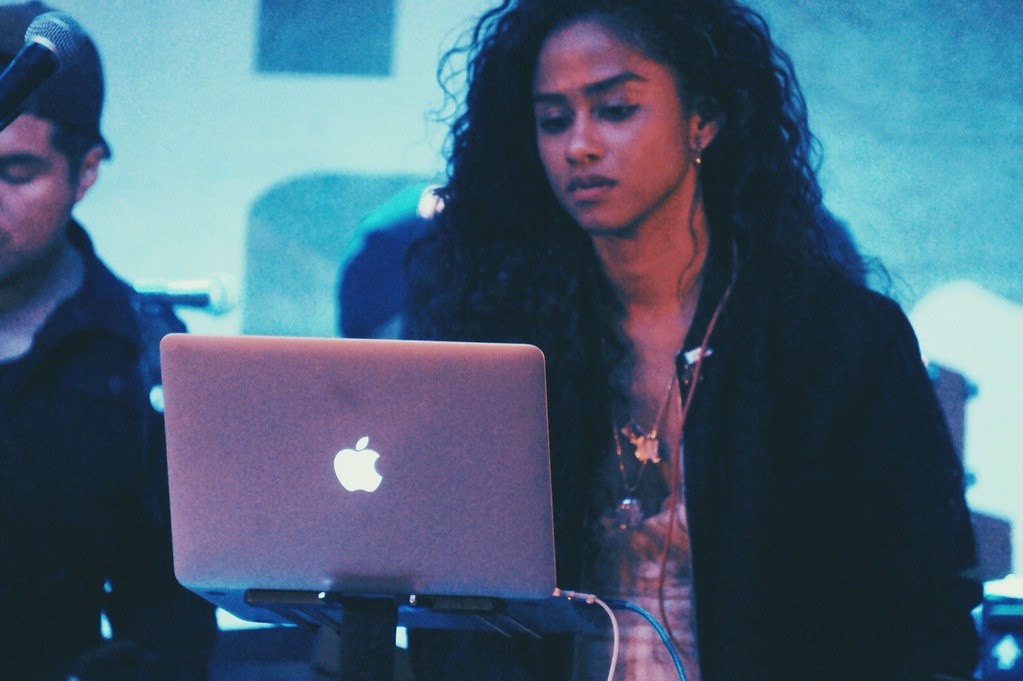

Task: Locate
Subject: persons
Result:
[0,3,220,681]
[402,1,984,678]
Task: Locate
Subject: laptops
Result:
[159,334,610,635]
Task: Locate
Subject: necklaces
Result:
[608,287,703,531]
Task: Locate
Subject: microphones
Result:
[0,12,86,133]
